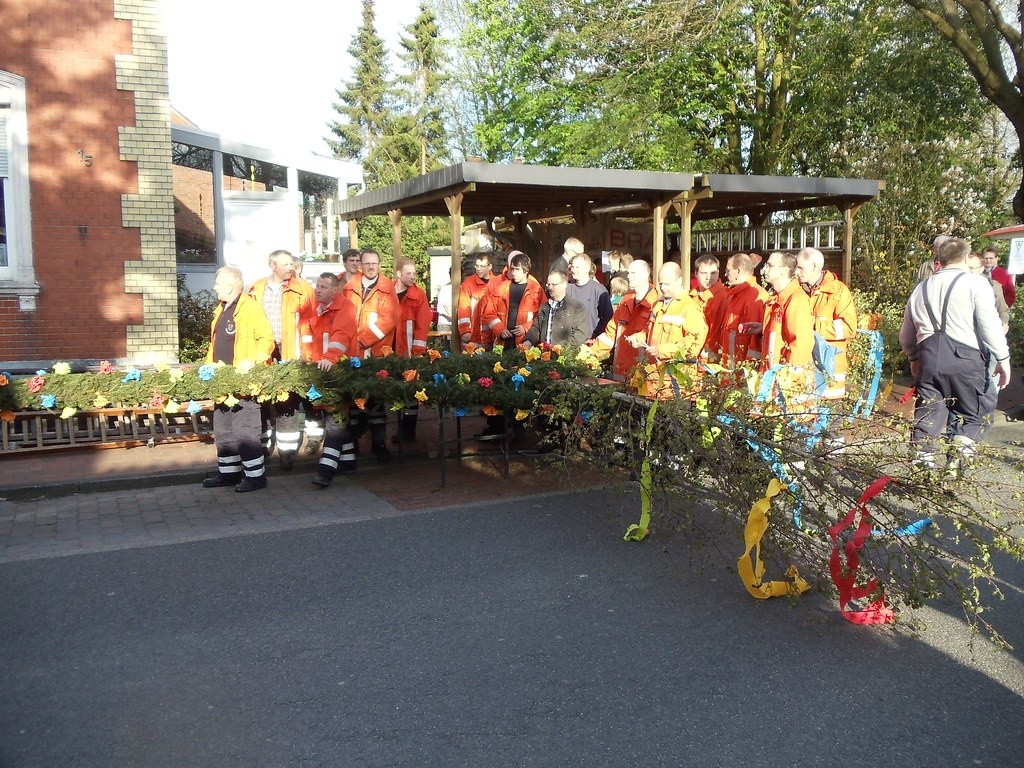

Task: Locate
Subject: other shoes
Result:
[203,474,240,487]
[473,425,505,441]
[371,440,390,455]
[235,478,266,492]
[265,456,272,468]
[512,429,524,443]
[332,463,356,474]
[280,454,293,469]
[304,437,323,454]
[391,431,415,443]
[312,473,331,486]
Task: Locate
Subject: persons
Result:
[437,266,463,329]
[898,239,1011,478]
[982,246,1015,308]
[248,249,315,469]
[294,249,430,463]
[202,266,267,492]
[946,252,1012,445]
[915,235,950,284]
[297,272,358,486]
[458,236,857,468]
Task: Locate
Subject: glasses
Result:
[763,262,783,268]
[474,265,489,269]
[545,282,561,287]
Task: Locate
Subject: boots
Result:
[909,451,943,494]
[942,436,976,486]
